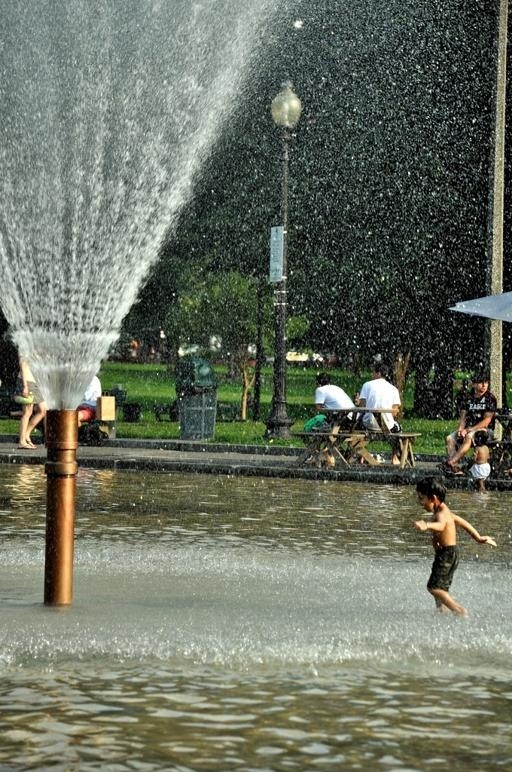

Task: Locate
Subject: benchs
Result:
[342,427,422,467]
[436,408,512,480]
[289,431,366,469]
[78,390,126,446]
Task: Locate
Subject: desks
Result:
[294,407,412,467]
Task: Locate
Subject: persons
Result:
[11,344,46,449]
[466,427,494,494]
[302,371,360,467]
[74,371,104,429]
[354,363,412,466]
[11,462,116,496]
[441,371,499,469]
[411,475,500,618]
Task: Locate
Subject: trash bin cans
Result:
[177,384,217,438]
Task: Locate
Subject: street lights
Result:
[266,80,303,440]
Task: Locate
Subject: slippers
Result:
[17,440,37,449]
